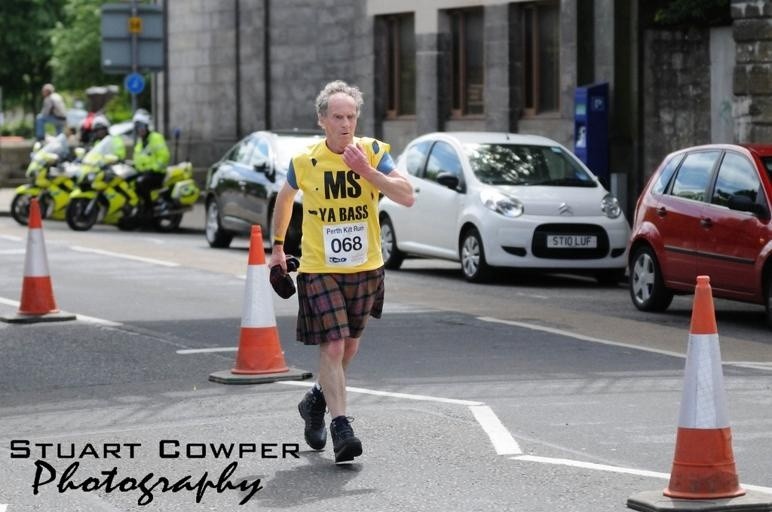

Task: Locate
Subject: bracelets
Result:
[271,237,284,245]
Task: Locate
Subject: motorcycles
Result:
[12,134,134,228]
[67,133,200,232]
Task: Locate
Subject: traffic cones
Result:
[626,275,772,512]
[208,225,315,384]
[0,196,77,324]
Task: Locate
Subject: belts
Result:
[54,116,65,120]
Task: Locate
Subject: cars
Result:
[377,130,635,282]
[627,141,772,319]
[203,130,360,254]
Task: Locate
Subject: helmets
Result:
[91,115,110,136]
[131,109,155,136]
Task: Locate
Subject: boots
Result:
[297,384,330,449]
[331,415,363,463]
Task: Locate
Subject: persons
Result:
[131,108,171,220]
[66,127,80,147]
[81,116,127,164]
[267,78,415,464]
[35,84,67,141]
